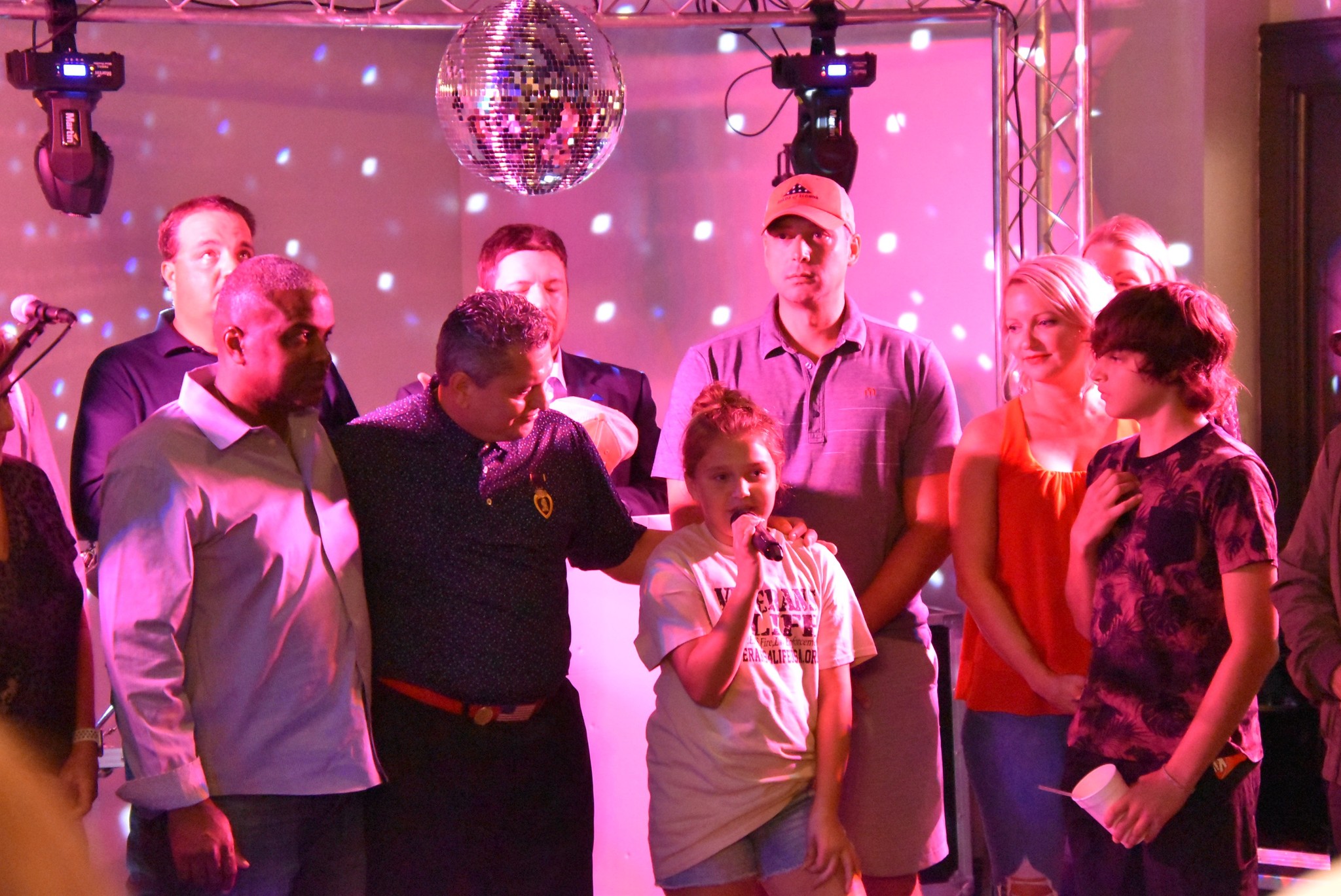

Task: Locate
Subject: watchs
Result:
[72,729,104,757]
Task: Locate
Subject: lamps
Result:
[772,1,877,192]
[4,1,126,220]
[433,0,630,195]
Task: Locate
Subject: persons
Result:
[0,173,1280,896]
[1269,423,1341,863]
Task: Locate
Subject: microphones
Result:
[10,294,76,325]
[730,511,783,561]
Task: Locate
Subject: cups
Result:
[1071,764,1147,849]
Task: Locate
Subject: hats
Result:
[763,173,857,235]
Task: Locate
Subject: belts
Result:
[382,677,543,722]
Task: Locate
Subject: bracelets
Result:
[1160,765,1195,794]
